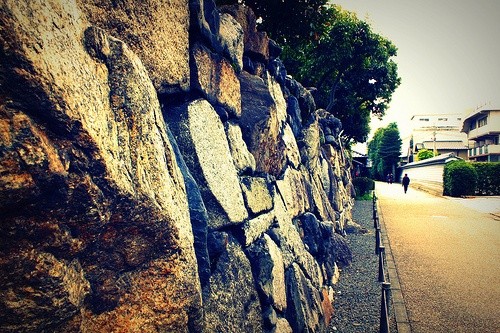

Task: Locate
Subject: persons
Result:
[402,174,410,194]
[387,173,393,184]
